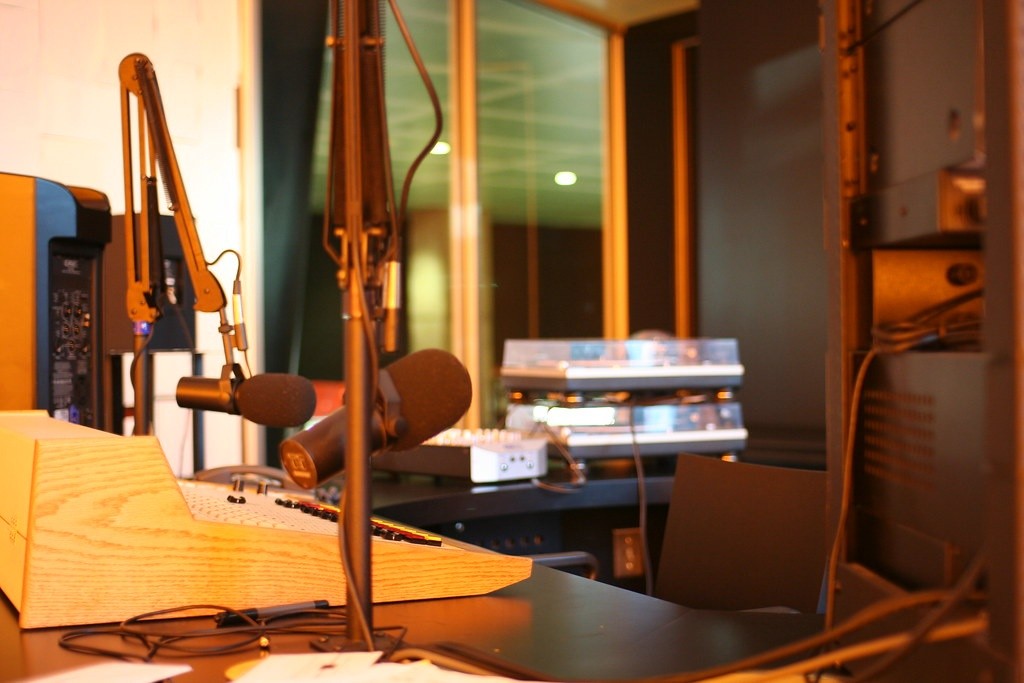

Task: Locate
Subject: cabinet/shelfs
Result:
[500,337,749,474]
[700,2,1024,658]
[345,449,835,594]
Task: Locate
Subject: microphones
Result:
[176,346,473,489]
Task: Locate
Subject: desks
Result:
[2,563,1021,683]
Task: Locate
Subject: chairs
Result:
[651,453,828,615]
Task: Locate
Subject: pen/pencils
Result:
[211,599,328,625]
